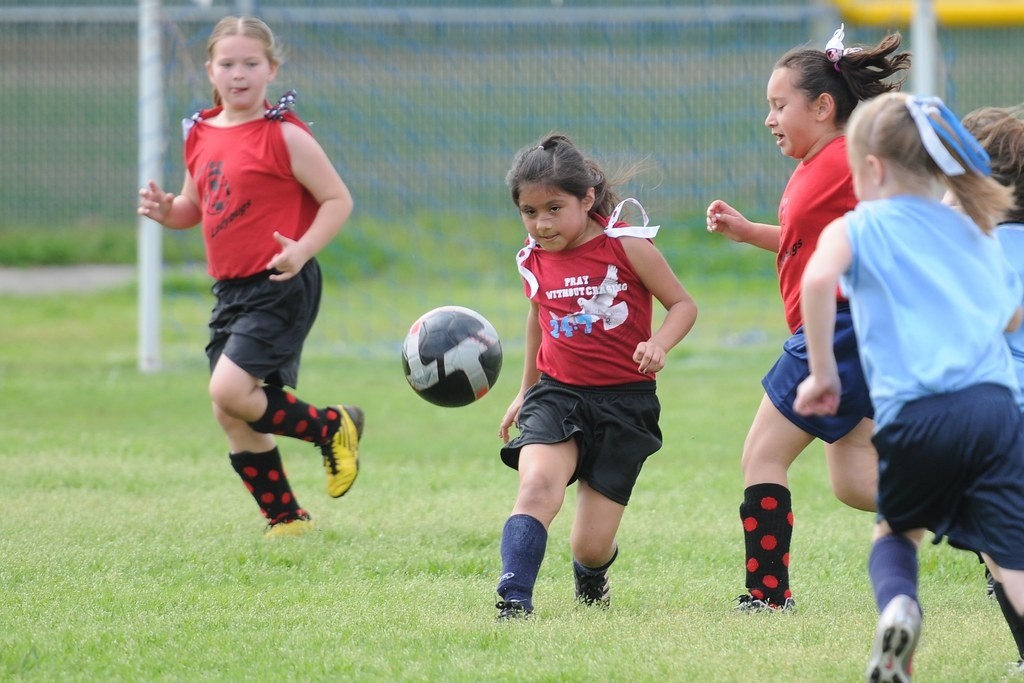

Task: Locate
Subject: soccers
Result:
[402,304,504,408]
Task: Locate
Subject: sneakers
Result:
[321,404,364,498]
[573,567,610,614]
[496,601,534,623]
[706,594,798,620]
[260,519,315,540]
[865,594,923,683]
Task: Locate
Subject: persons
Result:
[494,135,697,620]
[705,24,915,614]
[137,16,365,542]
[942,106,1023,669]
[793,91,1024,682]
